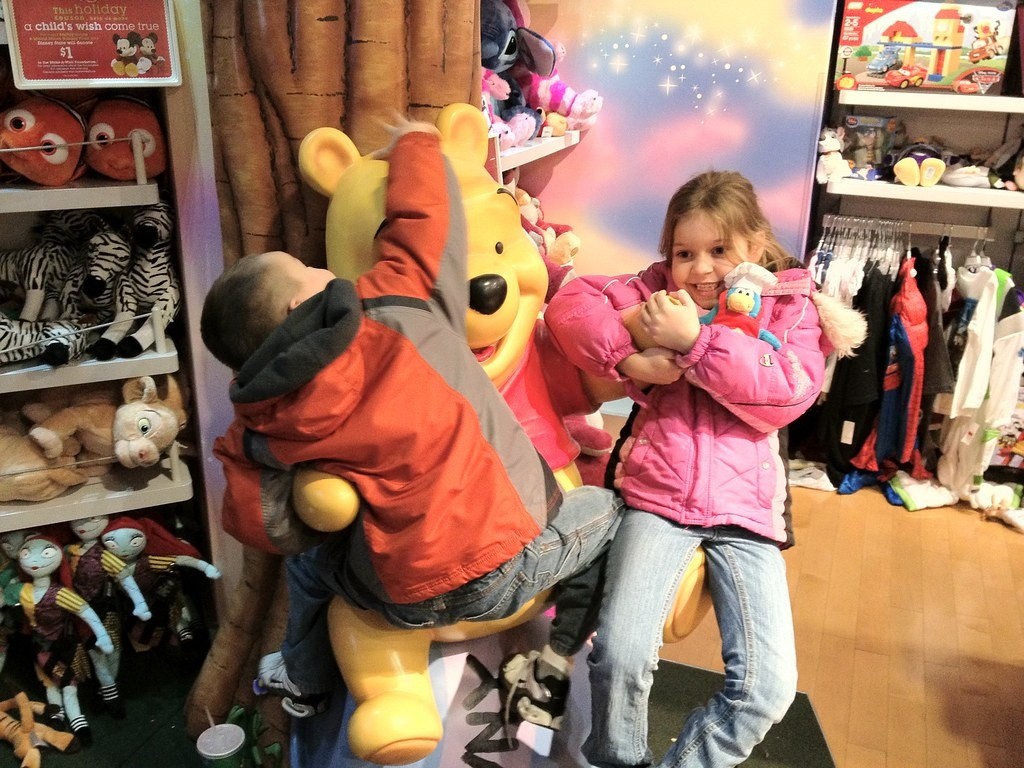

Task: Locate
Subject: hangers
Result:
[813,214,1017,290]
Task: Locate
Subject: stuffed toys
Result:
[480,0,603,151]
[0,87,166,186]
[816,121,1024,192]
[1,375,191,502]
[0,515,221,768]
[0,202,182,368]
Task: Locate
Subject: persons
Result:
[544,170,868,768]
[200,110,625,730]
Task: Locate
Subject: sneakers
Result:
[502,651,572,748]
[260,650,321,717]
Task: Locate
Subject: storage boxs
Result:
[831,1,1017,95]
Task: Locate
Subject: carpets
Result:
[0,618,839,768]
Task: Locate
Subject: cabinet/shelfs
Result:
[0,1,242,636]
[819,89,1023,212]
